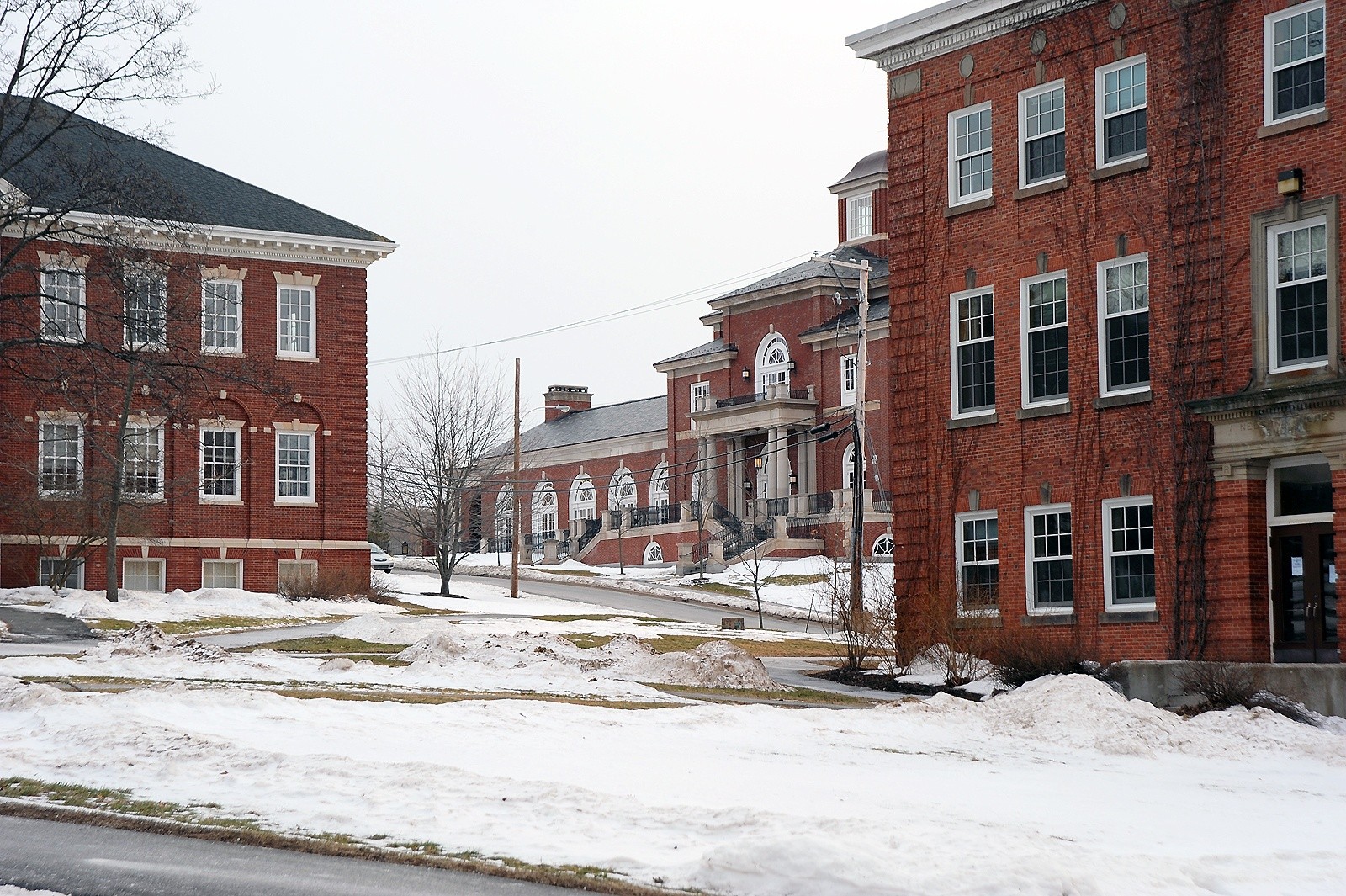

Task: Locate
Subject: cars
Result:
[368,541,395,573]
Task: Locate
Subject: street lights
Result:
[512,357,571,599]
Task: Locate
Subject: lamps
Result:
[753,454,763,471]
[741,366,752,386]
[787,357,797,377]
[1276,170,1302,196]
[743,477,753,495]
[789,470,799,492]
